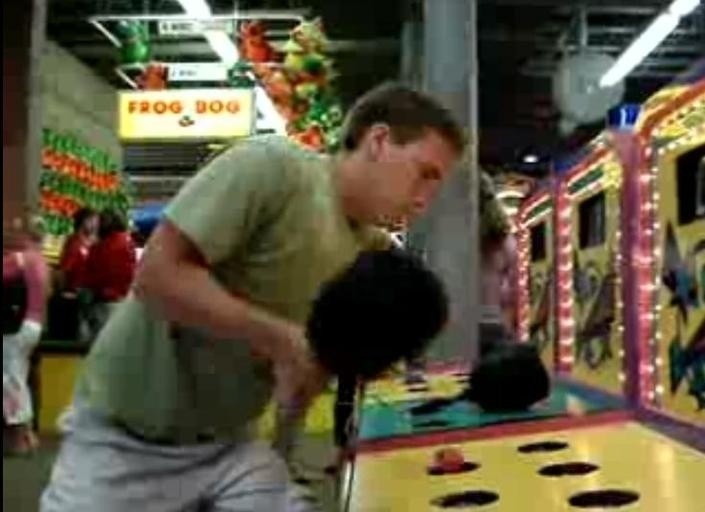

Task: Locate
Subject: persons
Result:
[480,171,521,358]
[73,205,139,329]
[36,77,470,512]
[3,213,54,455]
[56,205,100,344]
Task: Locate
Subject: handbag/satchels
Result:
[0,272,27,334]
